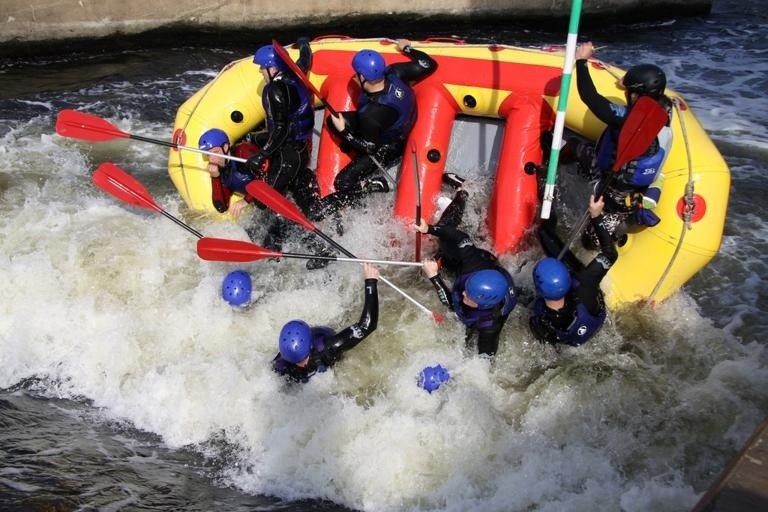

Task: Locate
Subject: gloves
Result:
[245,154,264,170]
[292,37,308,49]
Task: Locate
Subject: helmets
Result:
[352,49,385,81]
[417,365,449,394]
[199,128,229,150]
[465,269,509,310]
[623,63,666,98]
[279,320,313,363]
[253,45,289,67]
[532,257,571,300]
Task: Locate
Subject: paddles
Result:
[92,162,206,239]
[272,40,397,188]
[556,97,668,259]
[246,180,444,323]
[55,110,269,172]
[197,237,443,270]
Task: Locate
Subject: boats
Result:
[167,34,732,314]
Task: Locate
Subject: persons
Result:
[408,172,518,357]
[515,165,619,346]
[270,259,379,384]
[418,365,448,391]
[197,38,439,261]
[547,41,673,250]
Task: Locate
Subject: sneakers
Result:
[306,249,336,268]
[370,178,389,194]
[443,173,464,188]
